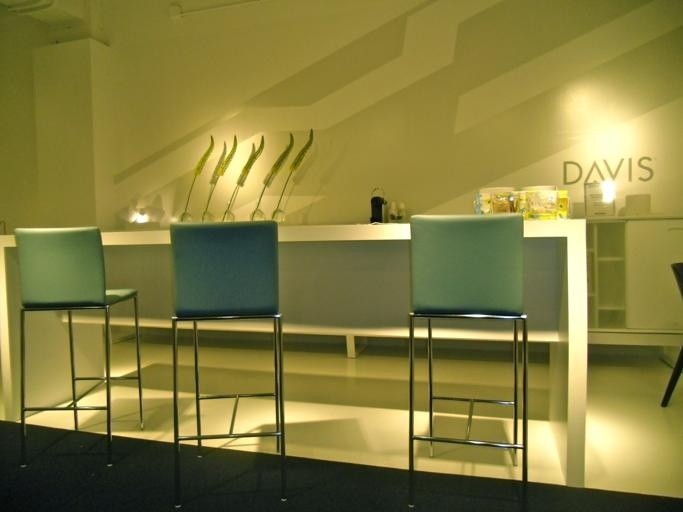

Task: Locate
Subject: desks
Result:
[1,216,590,489]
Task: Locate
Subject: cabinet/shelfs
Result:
[587,209,683,348]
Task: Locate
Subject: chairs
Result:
[169,219,287,504]
[13,224,144,470]
[405,214,532,496]
[660,258,683,408]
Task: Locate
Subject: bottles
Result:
[388,201,406,224]
